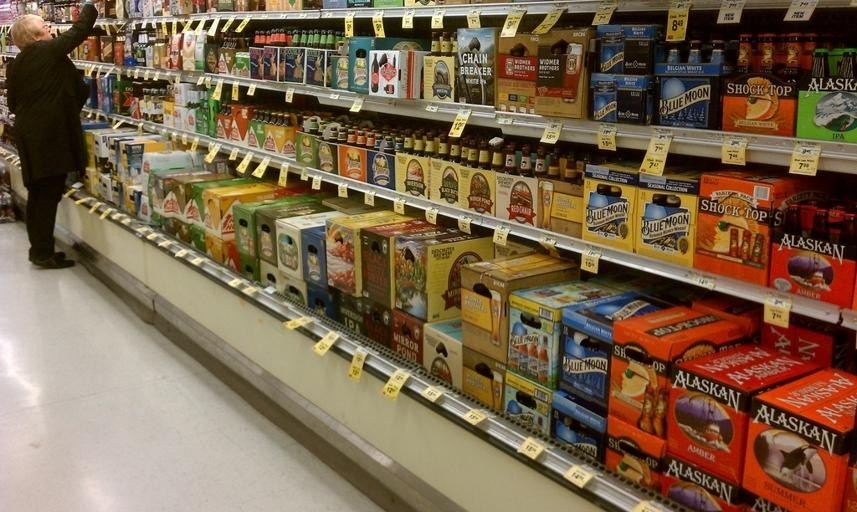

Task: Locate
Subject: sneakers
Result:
[29,249,74,268]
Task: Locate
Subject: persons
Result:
[4,1,101,269]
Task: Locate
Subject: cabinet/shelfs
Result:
[0,0,857,512]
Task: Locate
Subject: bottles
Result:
[637,382,668,439]
[508,335,549,384]
[113,23,857,262]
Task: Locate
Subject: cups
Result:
[489,289,501,346]
[490,371,504,410]
